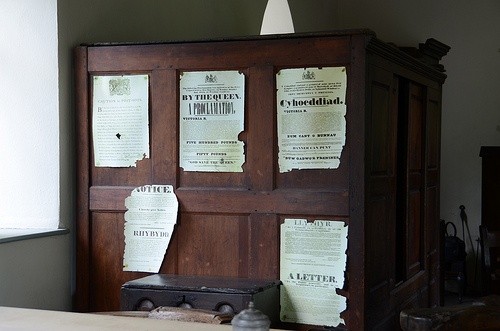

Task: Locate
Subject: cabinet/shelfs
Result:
[74,30,447,331]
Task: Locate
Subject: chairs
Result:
[478,224,499,294]
